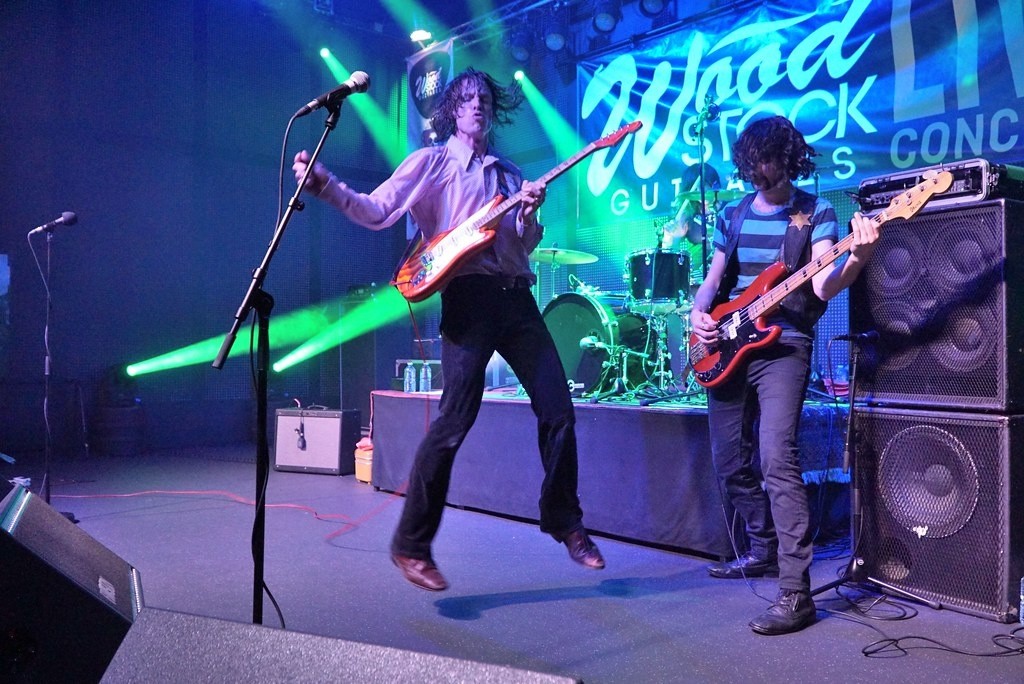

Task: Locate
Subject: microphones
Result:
[579,336,608,351]
[297,70,371,117]
[654,221,662,237]
[296,422,305,450]
[706,105,719,121]
[30,212,77,237]
[834,331,880,344]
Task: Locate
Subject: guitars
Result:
[396,120,642,304]
[688,168,954,388]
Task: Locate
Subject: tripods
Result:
[809,346,942,612]
[591,342,660,405]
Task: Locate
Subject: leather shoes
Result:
[390,550,447,591]
[562,528,605,569]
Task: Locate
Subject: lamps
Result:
[638,0,670,18]
[592,0,623,34]
[509,30,536,63]
[544,21,565,51]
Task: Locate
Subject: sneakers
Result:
[750,587,816,635]
[706,543,779,577]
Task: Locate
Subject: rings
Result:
[702,324,705,329]
[535,197,542,201]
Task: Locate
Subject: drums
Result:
[541,291,659,397]
[625,247,692,305]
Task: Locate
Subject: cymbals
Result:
[528,248,599,265]
[677,189,754,201]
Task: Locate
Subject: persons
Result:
[292,66,605,591]
[691,114,880,634]
[662,162,721,248]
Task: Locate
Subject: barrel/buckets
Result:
[88,365,145,459]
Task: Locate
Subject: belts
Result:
[444,274,531,292]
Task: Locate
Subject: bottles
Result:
[836,365,848,402]
[420,363,432,392]
[404,362,416,392]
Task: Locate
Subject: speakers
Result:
[845,196,1024,414]
[336,301,413,437]
[851,404,1024,626]
[272,407,362,474]
[0,484,145,684]
